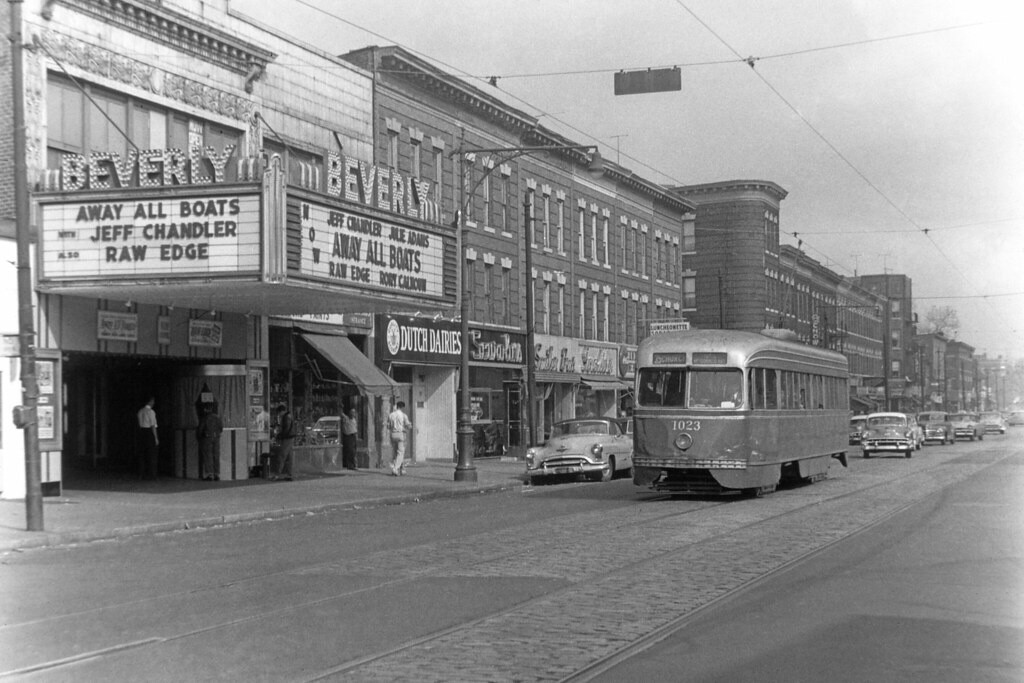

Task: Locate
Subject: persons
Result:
[271,405,295,476]
[137,398,159,479]
[386,401,412,476]
[342,408,359,470]
[196,413,222,482]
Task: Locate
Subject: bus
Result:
[632,329,851,497]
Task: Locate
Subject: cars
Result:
[979,412,1007,434]
[907,413,925,449]
[850,415,869,442]
[1009,411,1024,427]
[525,416,634,482]
[916,411,955,445]
[861,412,914,457]
[950,412,983,441]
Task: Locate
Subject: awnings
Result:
[582,381,628,391]
[850,397,873,411]
[302,334,400,398]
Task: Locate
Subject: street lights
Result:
[455,144,605,483]
[824,305,883,347]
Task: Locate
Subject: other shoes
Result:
[392,469,398,476]
[400,470,407,474]
[347,467,358,470]
[284,477,293,481]
[202,476,212,481]
[214,477,220,481]
